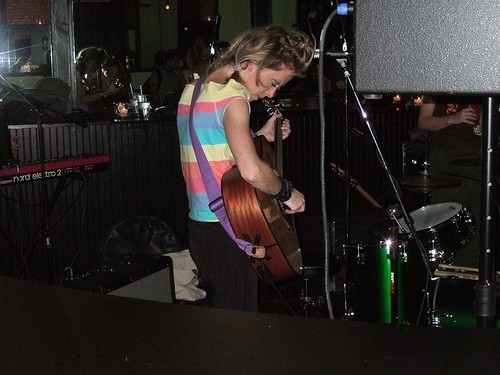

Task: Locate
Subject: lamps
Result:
[41,36,48,50]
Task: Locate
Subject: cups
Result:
[136,95,151,121]
[127,93,141,118]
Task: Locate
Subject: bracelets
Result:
[271,176,293,203]
[100,92,106,97]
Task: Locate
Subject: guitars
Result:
[329,162,383,208]
[221,96,304,280]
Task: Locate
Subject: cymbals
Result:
[433,164,500,184]
[393,174,463,188]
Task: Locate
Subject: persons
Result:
[417,96,484,208]
[177,25,314,313]
[0,109,22,164]
[140,38,231,111]
[75,46,130,113]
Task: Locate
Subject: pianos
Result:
[0,153,112,280]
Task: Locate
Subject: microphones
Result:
[47,174,66,210]
[327,162,359,188]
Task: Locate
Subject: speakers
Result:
[65,255,176,305]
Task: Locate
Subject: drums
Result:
[395,201,474,263]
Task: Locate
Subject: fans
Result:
[98,214,176,282]
